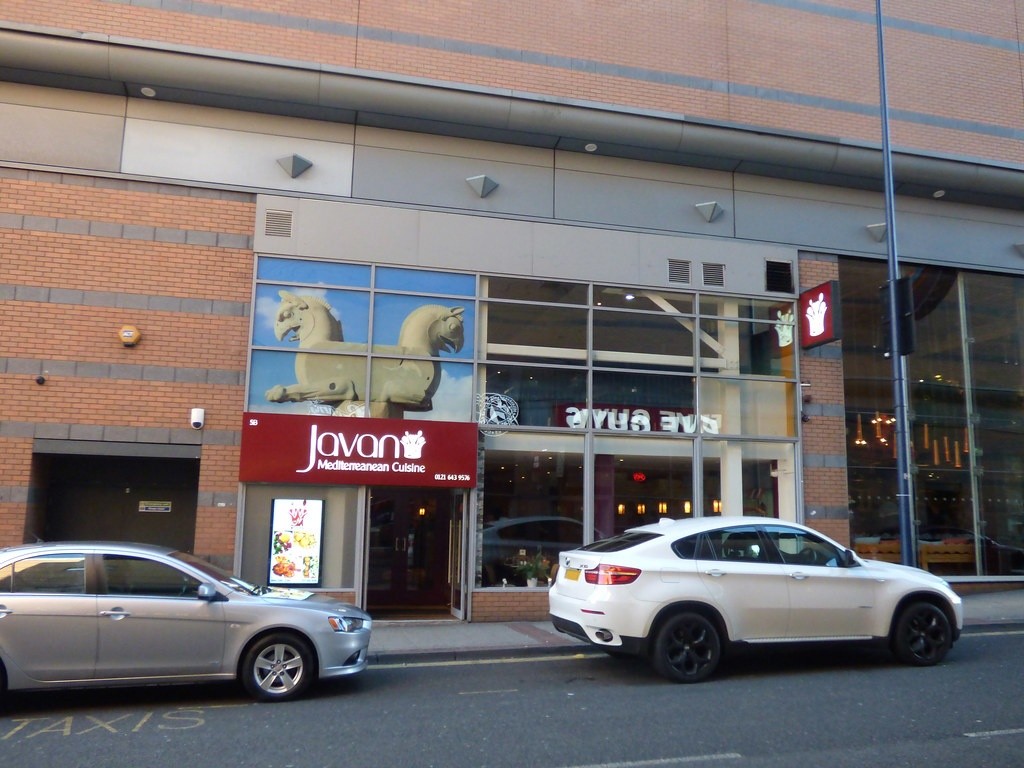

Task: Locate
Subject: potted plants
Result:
[513,551,545,587]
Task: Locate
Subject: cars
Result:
[548,513,964,680]
[482,516,609,570]
[0,541,375,708]
